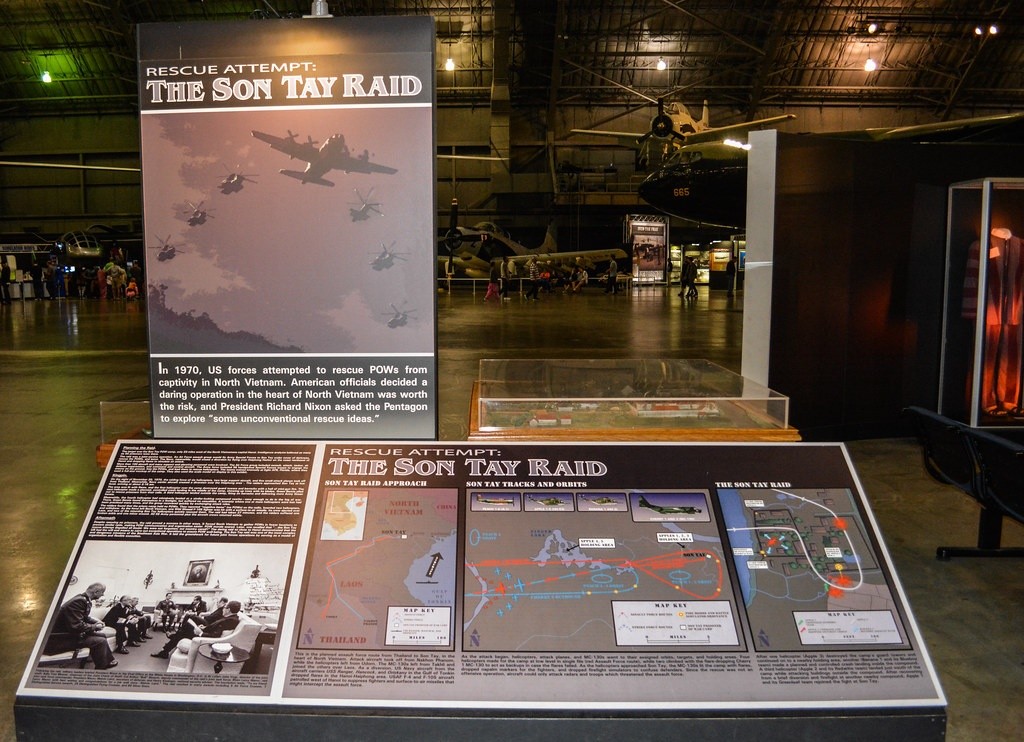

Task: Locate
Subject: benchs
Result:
[904,404,1024,562]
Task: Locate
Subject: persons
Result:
[667,258,676,288]
[0,258,143,307]
[727,257,737,296]
[678,254,698,299]
[483,255,588,302]
[601,253,618,296]
[44,582,240,676]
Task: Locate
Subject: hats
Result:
[103,263,114,273]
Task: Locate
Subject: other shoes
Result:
[523,294,530,302]
[533,298,540,302]
[484,298,489,303]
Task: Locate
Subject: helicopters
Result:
[365,240,410,272]
[150,233,185,262]
[214,158,261,197]
[382,300,417,330]
[183,199,218,228]
[346,186,386,223]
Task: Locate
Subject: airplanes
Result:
[438,197,629,295]
[0,223,144,273]
[570,84,798,181]
[252,129,399,188]
